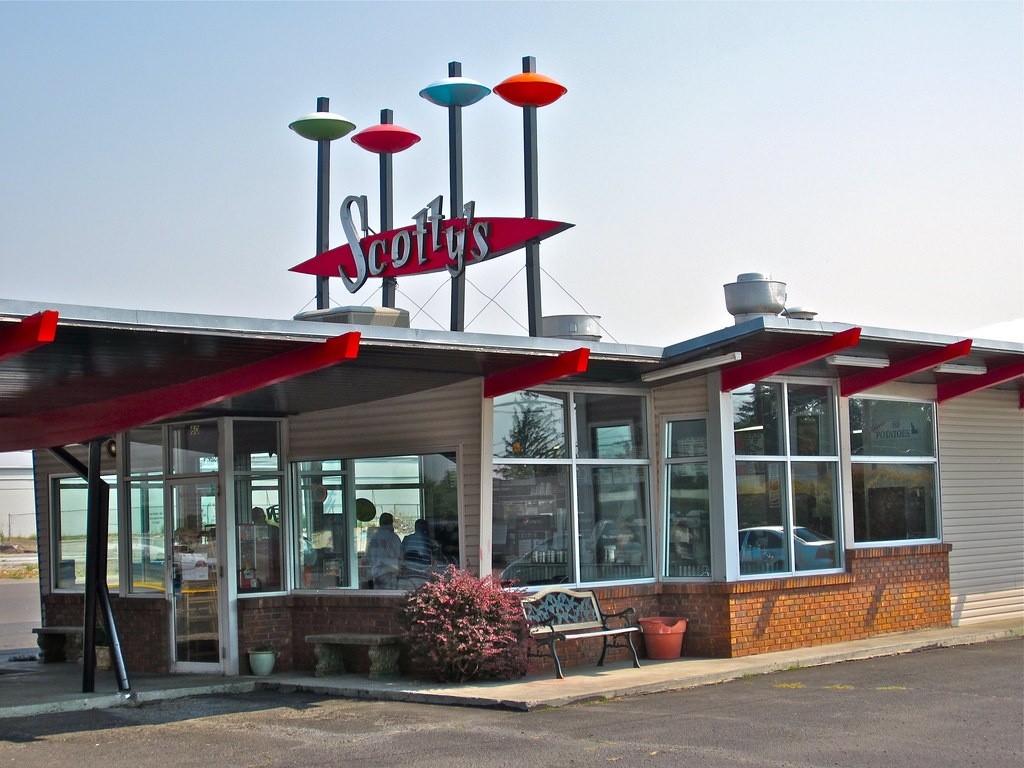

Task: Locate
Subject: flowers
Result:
[395,563,536,683]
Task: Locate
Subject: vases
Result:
[638,616,690,660]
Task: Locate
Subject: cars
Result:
[497,528,634,587]
[738,524,837,573]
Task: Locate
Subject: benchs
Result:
[305,634,407,681]
[521,587,643,680]
[33,627,86,664]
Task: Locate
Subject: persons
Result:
[398,519,442,591]
[367,513,402,589]
[250,507,267,525]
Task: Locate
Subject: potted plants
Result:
[94,629,111,670]
[247,641,280,675]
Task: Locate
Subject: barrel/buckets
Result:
[638,617,689,660]
